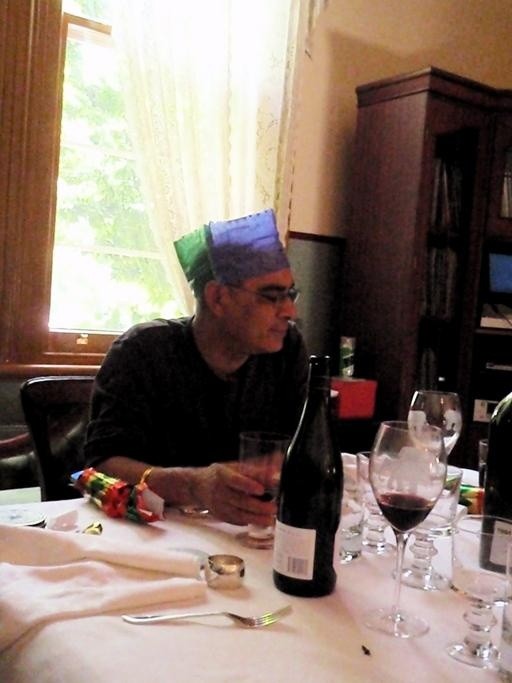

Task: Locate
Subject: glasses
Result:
[224,282,301,309]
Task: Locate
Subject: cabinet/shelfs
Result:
[342,94,510,461]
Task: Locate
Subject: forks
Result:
[121,603,295,629]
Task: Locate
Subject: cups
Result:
[477,437,489,488]
[331,498,364,567]
[407,388,463,459]
[204,553,245,590]
[495,541,512,682]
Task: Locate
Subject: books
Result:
[479,303,512,329]
[500,163,512,218]
[411,159,461,412]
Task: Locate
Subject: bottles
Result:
[271,352,339,600]
[476,391,512,574]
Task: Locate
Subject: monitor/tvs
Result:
[484,237,512,305]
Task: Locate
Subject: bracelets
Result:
[136,466,154,484]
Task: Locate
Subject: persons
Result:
[83,209,310,527]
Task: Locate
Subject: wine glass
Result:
[390,462,463,592]
[236,429,292,548]
[363,420,448,640]
[354,451,393,556]
[446,514,512,669]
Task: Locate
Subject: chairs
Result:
[20,379,94,503]
